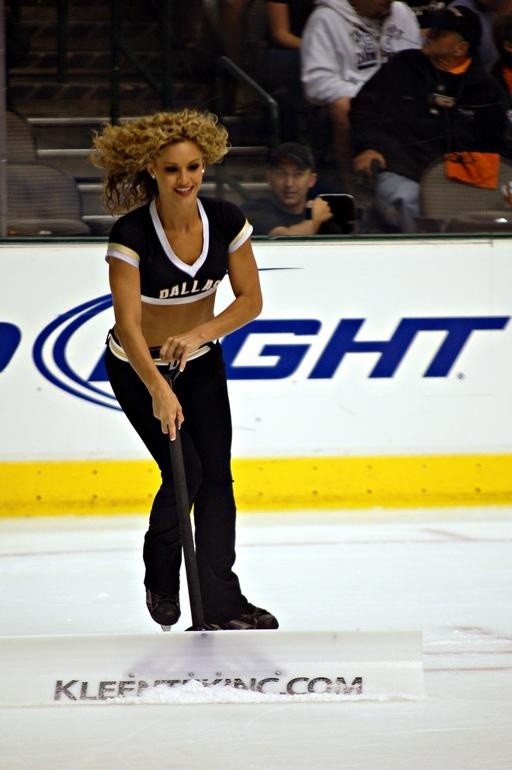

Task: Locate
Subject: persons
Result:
[84,105,281,629]
[211,0,512,242]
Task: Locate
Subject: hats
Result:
[415,4,484,52]
[260,141,317,173]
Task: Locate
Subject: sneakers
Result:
[145,572,181,625]
[204,603,280,628]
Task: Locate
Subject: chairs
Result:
[0,0,512,242]
